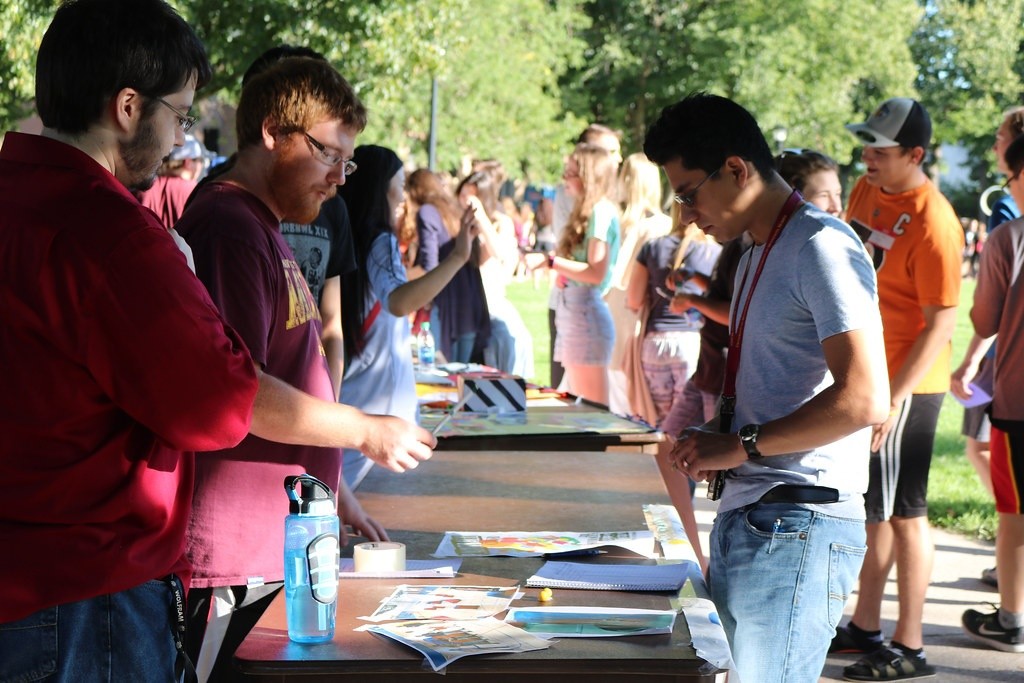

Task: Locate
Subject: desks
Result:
[236,448,738,683]
[413,363,666,454]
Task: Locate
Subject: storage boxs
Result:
[456,370,526,415]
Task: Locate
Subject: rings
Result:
[682,460,691,468]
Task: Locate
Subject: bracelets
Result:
[548,251,555,269]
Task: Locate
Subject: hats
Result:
[164,134,202,163]
[846,98,931,147]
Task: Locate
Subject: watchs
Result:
[737,424,764,458]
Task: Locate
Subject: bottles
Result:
[284,473,339,643]
[417,322,435,368]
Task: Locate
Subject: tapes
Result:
[352,541,407,574]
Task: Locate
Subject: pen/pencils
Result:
[542,548,608,559]
[431,382,481,436]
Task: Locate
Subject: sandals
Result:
[841,645,936,683]
[828,618,885,653]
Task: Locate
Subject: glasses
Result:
[297,128,357,176]
[779,147,809,180]
[145,95,198,132]
[674,164,723,207]
[1001,172,1020,195]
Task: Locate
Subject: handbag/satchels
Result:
[622,336,658,428]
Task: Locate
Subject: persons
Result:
[164,56,440,683]
[0,0,436,683]
[836,97,964,683]
[135,47,1024,651]
[627,90,892,683]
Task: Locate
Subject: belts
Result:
[759,486,840,503]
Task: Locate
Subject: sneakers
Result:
[962,609,1024,651]
[983,565,997,586]
